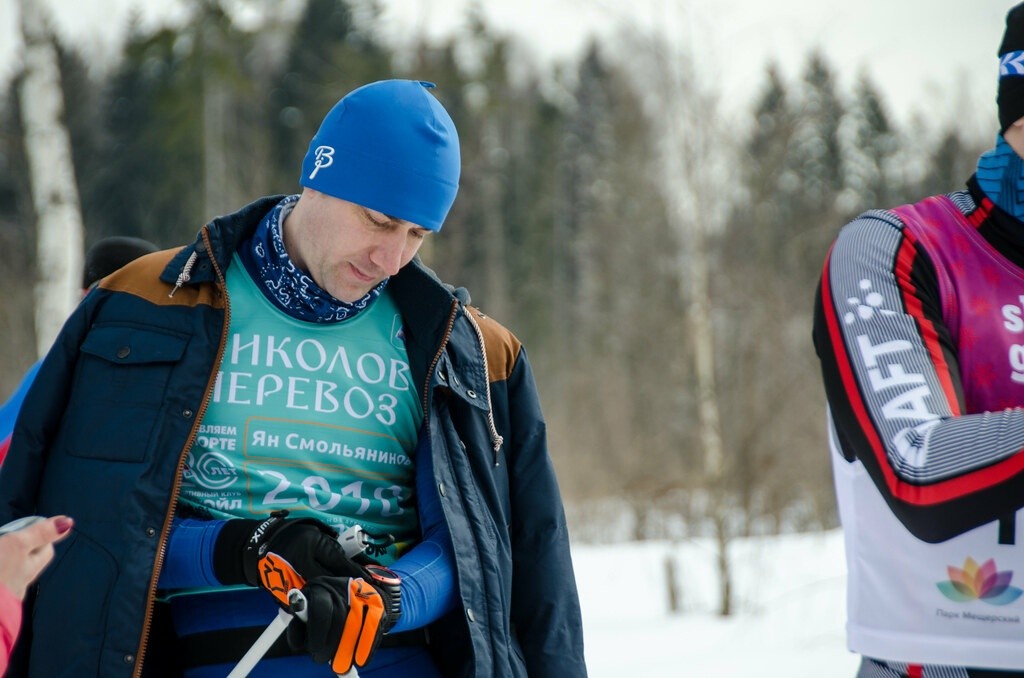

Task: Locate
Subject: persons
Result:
[0,80,587,678]
[1,237,163,467]
[0,516,73,678]
[813,3,1024,678]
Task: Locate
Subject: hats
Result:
[82,236,162,287]
[996,2,1024,129]
[300,79,461,234]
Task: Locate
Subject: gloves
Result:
[246,508,366,612]
[285,574,390,676]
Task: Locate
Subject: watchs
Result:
[365,565,402,634]
